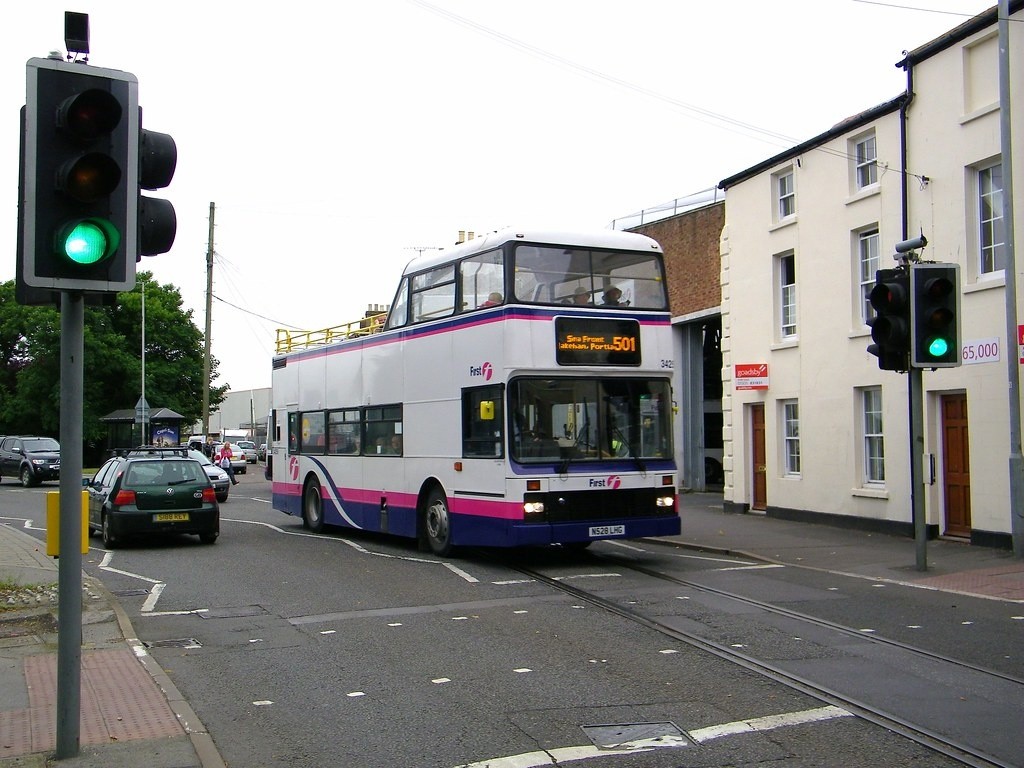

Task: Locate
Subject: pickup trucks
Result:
[0,435,61,487]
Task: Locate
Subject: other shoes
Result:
[233,481,239,485]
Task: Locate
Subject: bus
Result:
[271,226,681,558]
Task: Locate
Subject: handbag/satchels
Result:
[221,456,229,468]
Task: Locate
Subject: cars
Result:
[257,443,266,462]
[82,447,220,549]
[213,444,247,474]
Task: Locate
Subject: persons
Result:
[203,437,216,464]
[318,418,401,454]
[643,417,654,456]
[511,397,539,442]
[561,287,592,305]
[600,284,627,307]
[480,292,502,308]
[374,315,386,333]
[219,441,240,485]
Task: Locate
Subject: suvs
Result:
[128,445,230,503]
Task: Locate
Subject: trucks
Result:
[188,435,206,447]
[219,430,250,445]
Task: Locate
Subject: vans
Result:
[236,440,257,464]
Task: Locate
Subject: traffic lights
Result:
[131,104,177,263]
[866,267,911,371]
[23,56,137,292]
[911,262,962,368]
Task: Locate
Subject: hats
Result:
[573,287,590,301]
[602,285,622,301]
